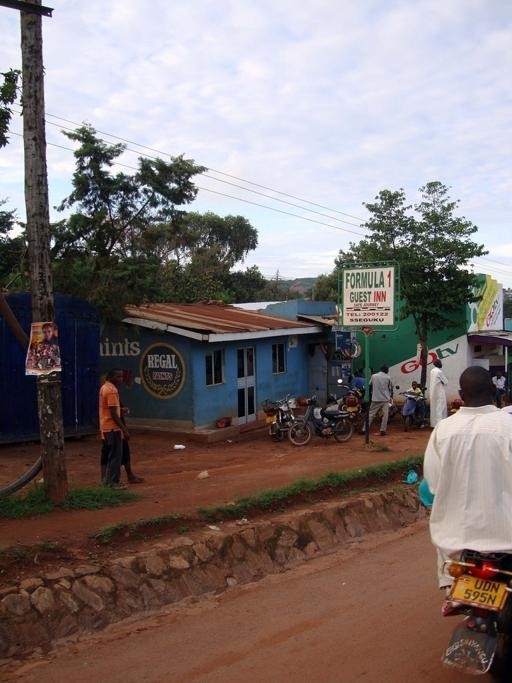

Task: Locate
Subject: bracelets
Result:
[123,427,127,432]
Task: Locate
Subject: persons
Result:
[25,322,60,370]
[98,367,130,489]
[100,372,144,483]
[357,364,394,435]
[491,368,506,406]
[351,368,365,386]
[406,381,425,418]
[422,365,512,617]
[429,358,449,428]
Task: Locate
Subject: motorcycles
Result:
[262,378,364,447]
[394,385,427,432]
[441,549,512,683]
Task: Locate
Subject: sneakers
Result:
[112,480,128,490]
[441,597,472,618]
[360,430,386,436]
